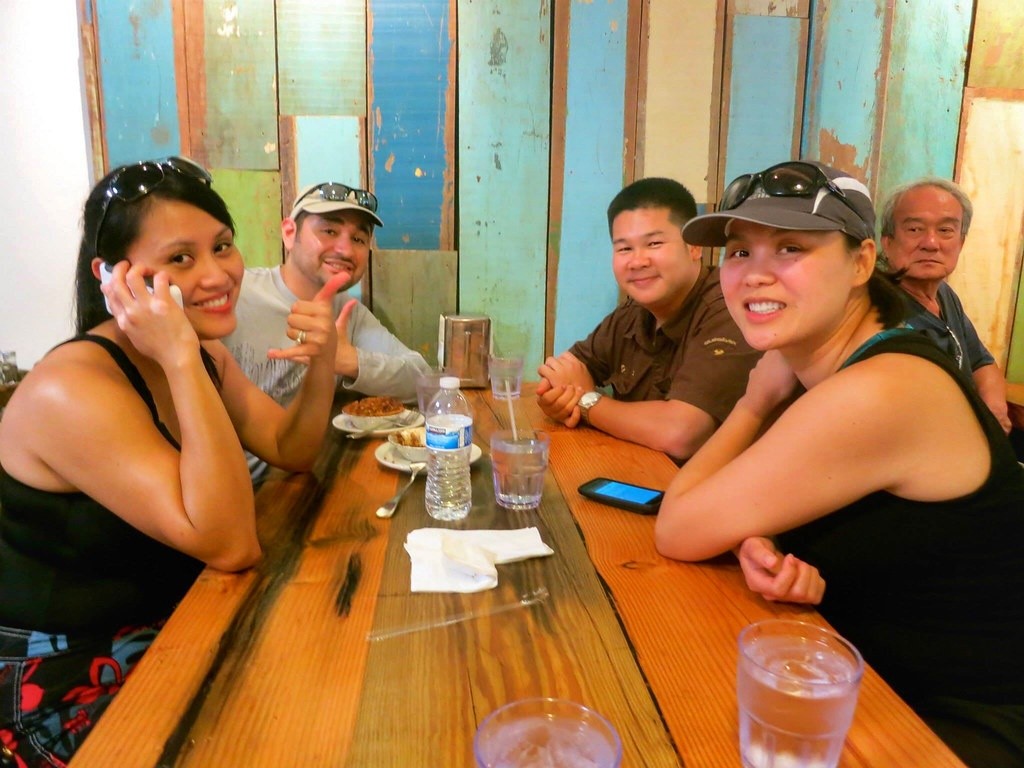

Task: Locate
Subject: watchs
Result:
[575,391,605,427]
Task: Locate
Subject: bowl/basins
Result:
[388,428,429,462]
[342,404,404,430]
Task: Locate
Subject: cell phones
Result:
[100,262,183,316]
[577,477,665,514]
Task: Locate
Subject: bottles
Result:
[425,377,473,520]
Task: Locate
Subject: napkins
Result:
[409,532,499,594]
[405,526,556,568]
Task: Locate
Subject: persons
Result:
[0,159,349,768]
[534,178,764,467]
[876,178,1024,462]
[653,151,1024,767]
[224,182,434,494]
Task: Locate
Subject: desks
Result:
[63,380,967,768]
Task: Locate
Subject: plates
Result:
[375,441,482,474]
[332,410,425,439]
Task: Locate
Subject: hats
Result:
[290,186,385,230]
[681,160,875,243]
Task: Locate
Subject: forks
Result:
[347,408,420,439]
[376,463,427,518]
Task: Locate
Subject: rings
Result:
[295,329,303,344]
[302,331,306,342]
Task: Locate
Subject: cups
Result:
[474,698,621,768]
[416,370,460,417]
[490,431,552,510]
[0,351,18,386]
[488,352,523,400]
[739,619,864,768]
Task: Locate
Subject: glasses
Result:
[94,156,212,257]
[722,161,868,227]
[294,181,379,214]
